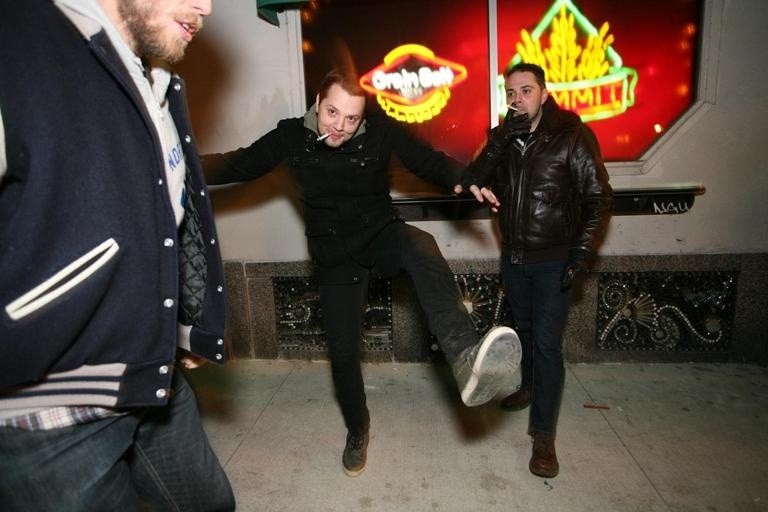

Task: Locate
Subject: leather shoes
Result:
[502,391,530,408]
[529,435,559,476]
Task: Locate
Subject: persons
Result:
[470,63,613,479]
[197,63,523,476]
[0,0,239,511]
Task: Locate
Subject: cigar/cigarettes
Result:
[317,132,332,141]
[505,104,518,111]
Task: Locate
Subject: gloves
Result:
[488,102,530,147]
[559,246,595,292]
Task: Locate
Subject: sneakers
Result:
[343,426,369,476]
[454,327,522,407]
